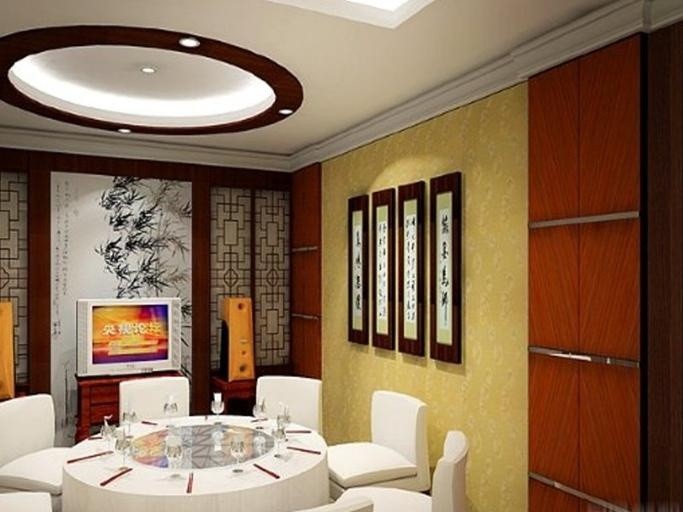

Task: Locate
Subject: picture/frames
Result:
[345,171,462,365]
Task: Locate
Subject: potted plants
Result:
[345,171,462,365]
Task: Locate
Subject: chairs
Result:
[119,376,189,426]
[327,390,431,500]
[311,499,373,511]
[256,376,322,434]
[337,430,468,510]
[1,492,52,511]
[1,394,70,509]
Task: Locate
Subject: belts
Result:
[64,400,322,495]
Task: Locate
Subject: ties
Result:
[76,297,182,378]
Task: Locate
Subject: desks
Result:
[62,415,327,511]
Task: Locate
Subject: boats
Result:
[0,301,14,400]
[216,294,255,382]
